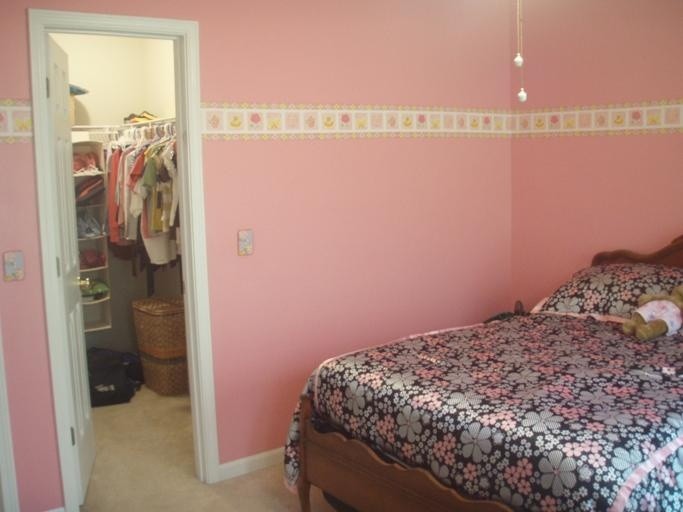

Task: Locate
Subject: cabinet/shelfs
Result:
[72,139,120,335]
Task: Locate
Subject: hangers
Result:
[115,123,176,148]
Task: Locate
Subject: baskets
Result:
[129,292,191,398]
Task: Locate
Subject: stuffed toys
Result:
[620,283,682,340]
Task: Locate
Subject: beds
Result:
[294,228,683,512]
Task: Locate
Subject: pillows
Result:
[530,259,683,325]
